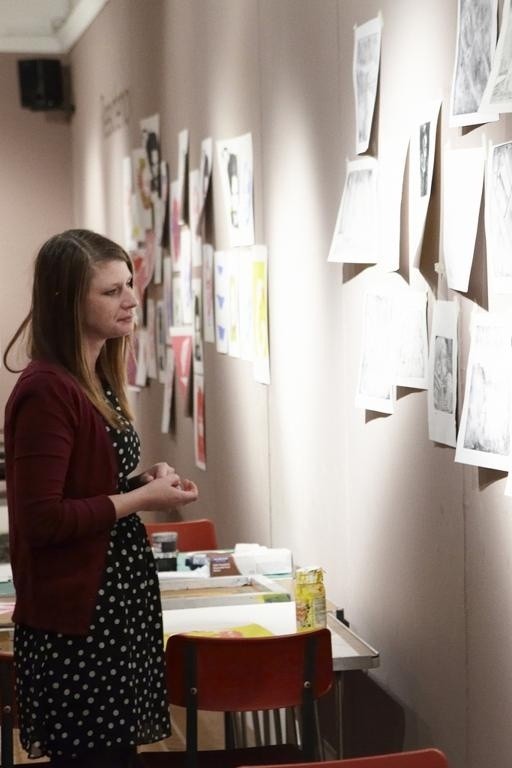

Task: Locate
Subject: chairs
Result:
[0,652,19,760]
[144,518,215,550]
[163,628,329,762]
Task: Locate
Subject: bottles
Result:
[151,531,177,572]
[295,567,327,635]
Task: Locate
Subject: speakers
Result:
[18,59,63,109]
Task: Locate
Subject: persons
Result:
[3,231,199,766]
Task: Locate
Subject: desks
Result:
[1,548,381,760]
[1,552,300,652]
